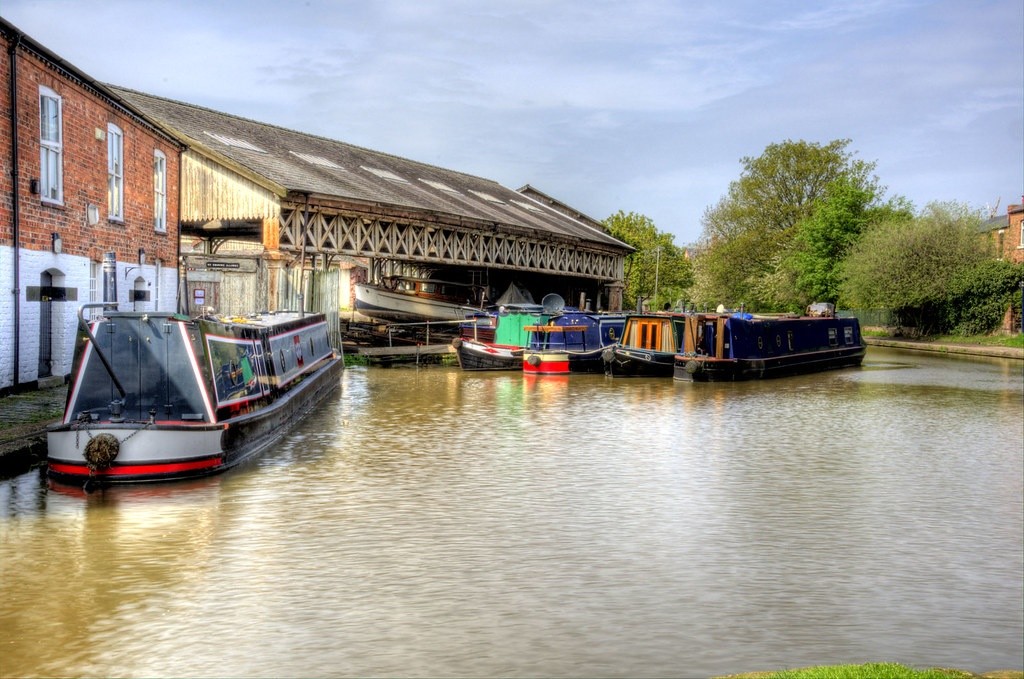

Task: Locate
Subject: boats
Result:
[603,313,688,377]
[454,307,563,371]
[44,306,345,485]
[353,275,488,328]
[523,310,628,376]
[675,312,869,380]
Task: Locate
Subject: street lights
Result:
[655,245,663,313]
